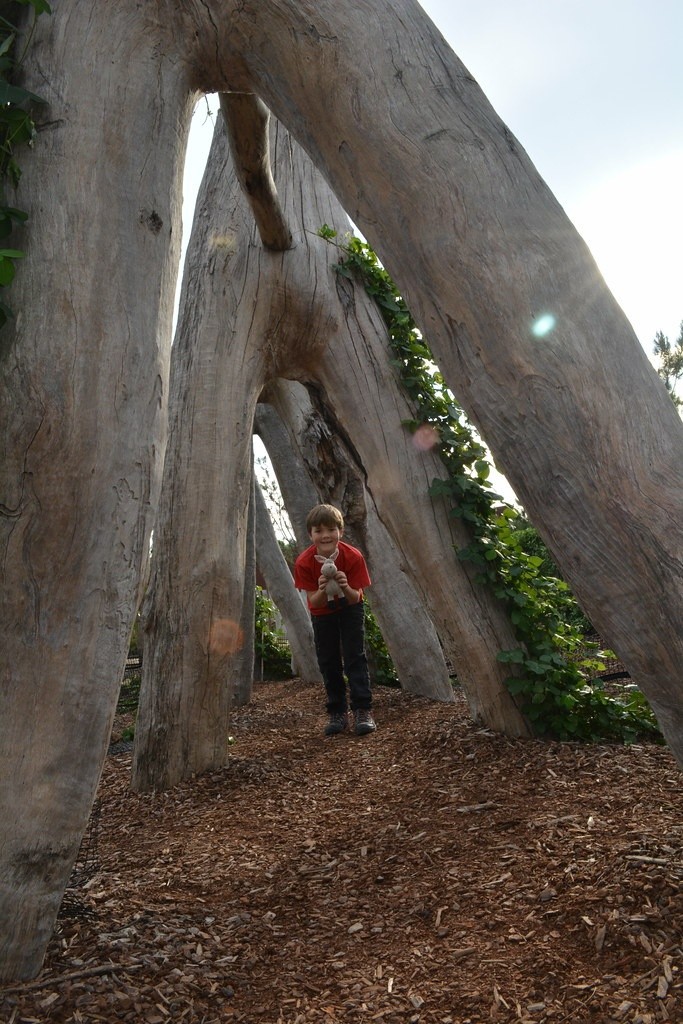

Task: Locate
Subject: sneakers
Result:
[352,708,375,735]
[325,711,349,735]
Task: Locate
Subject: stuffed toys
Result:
[314,550,347,609]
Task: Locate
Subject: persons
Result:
[294,504,377,735]
